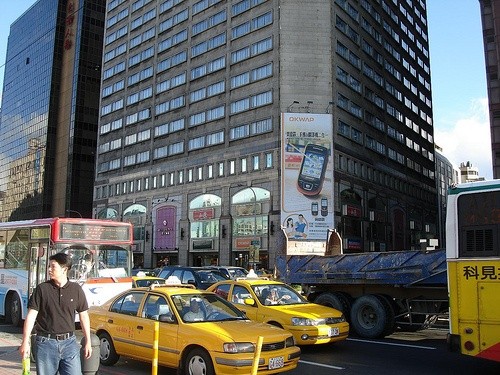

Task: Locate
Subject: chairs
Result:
[237,293,252,304]
[152,303,170,320]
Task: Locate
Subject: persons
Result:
[19,252,93,375]
[183,298,217,321]
[265,288,292,305]
[78,254,95,277]
[257,268,266,275]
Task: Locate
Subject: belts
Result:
[37,331,73,341]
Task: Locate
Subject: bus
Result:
[444,178,500,362]
[0,217,134,329]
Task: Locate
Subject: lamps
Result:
[288,101,300,112]
[305,101,313,112]
[326,101,334,113]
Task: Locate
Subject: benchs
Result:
[118,301,155,315]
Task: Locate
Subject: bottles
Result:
[22,352,30,375]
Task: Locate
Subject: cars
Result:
[129,265,269,308]
[87,275,301,375]
[203,268,350,345]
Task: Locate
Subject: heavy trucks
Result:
[275,248,448,340]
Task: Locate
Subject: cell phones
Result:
[297,143,328,196]
[311,202,318,216]
[321,199,328,216]
